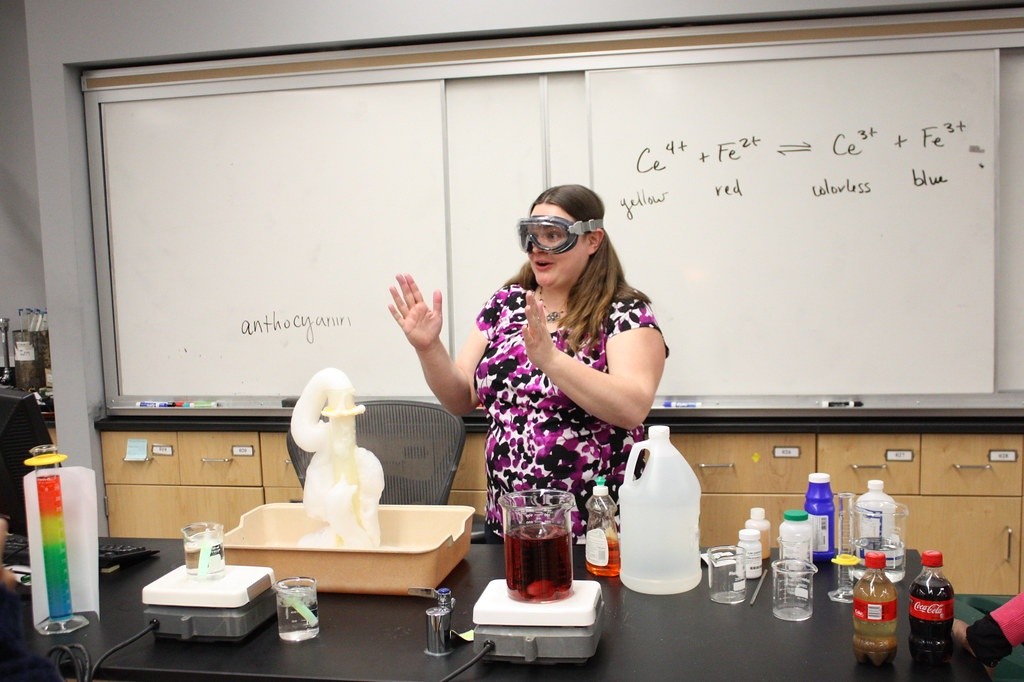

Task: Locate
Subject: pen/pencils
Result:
[9,568,30,574]
[21,575,31,583]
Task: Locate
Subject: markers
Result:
[478,404,483,407]
[175,401,221,407]
[136,402,166,407]
[662,402,701,408]
[141,401,176,406]
[823,401,864,408]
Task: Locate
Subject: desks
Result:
[24,537,995,682]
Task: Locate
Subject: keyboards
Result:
[1,534,160,563]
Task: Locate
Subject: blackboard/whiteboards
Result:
[445,71,546,363]
[101,79,454,396]
[543,71,589,190]
[585,48,999,395]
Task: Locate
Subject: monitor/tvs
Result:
[0,387,60,536]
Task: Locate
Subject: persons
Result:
[390,185,669,543]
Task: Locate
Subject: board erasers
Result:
[282,398,298,407]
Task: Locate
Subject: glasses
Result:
[518,215,577,255]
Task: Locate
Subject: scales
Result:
[140,564,277,639]
[470,579,605,661]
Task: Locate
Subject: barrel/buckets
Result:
[12,328,50,391]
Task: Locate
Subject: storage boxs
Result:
[221,502,475,595]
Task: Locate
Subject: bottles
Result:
[804,473,835,561]
[735,529,762,579]
[618,425,703,595]
[745,508,771,559]
[909,549,955,666]
[779,510,812,564]
[856,479,896,538]
[852,552,899,666]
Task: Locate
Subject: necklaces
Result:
[540,285,568,322]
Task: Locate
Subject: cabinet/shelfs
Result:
[432,431,488,515]
[816,433,1024,596]
[101,429,264,538]
[642,433,816,548]
[260,432,436,505]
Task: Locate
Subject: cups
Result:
[497,489,576,604]
[180,522,226,582]
[847,501,909,583]
[272,576,318,641]
[771,558,818,621]
[707,545,746,604]
[776,535,811,563]
